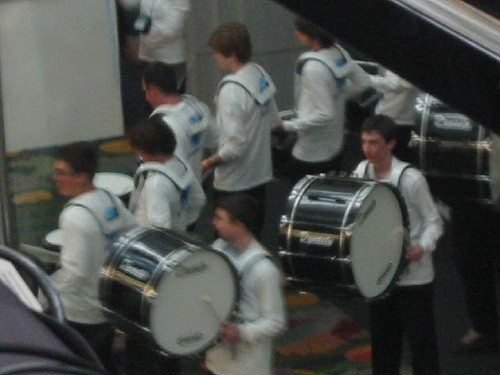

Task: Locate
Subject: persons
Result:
[201,193,286,375]
[141,62,217,233]
[37,141,138,357]
[275,17,370,181]
[119,0,193,129]
[202,21,282,245]
[369,69,416,161]
[127,117,206,233]
[350,114,445,375]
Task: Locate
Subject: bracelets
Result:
[202,160,210,171]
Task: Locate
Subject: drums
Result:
[136,153,144,166]
[97,224,241,357]
[278,173,411,302]
[269,109,298,150]
[407,90,492,205]
[91,171,134,210]
[43,229,63,252]
